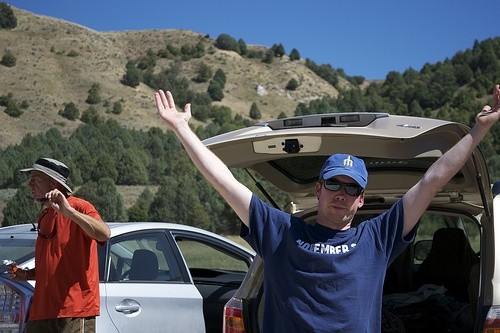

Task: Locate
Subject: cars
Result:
[202,111,500,333]
[0,222,256,333]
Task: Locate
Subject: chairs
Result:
[414,227,480,310]
[129,249,158,281]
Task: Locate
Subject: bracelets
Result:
[21,267,31,281]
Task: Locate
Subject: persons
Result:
[154,84,500,333]
[5,157,111,333]
